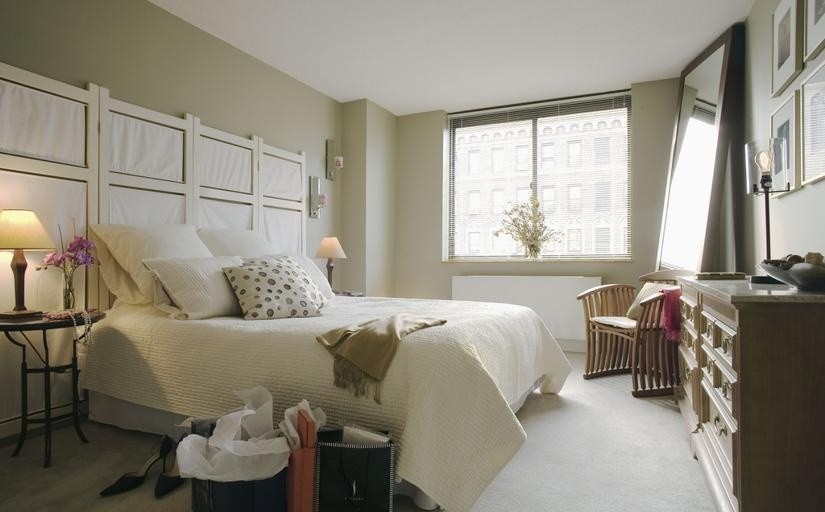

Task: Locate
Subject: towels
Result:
[659,289,682,343]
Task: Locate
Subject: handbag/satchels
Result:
[287,429,395,511]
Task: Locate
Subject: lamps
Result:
[0,209,57,323]
[328,156,344,181]
[745,138,790,284]
[313,194,328,218]
[313,236,347,289]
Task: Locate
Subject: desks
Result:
[1,309,106,468]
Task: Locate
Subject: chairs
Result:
[576,270,702,398]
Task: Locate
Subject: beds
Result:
[77,297,571,512]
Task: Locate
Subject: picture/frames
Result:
[803,0,825,64]
[799,61,825,187]
[769,90,802,199]
[769,0,803,99]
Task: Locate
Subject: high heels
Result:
[155,433,188,498]
[99,434,172,497]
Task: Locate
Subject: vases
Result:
[60,268,75,311]
[527,241,543,263]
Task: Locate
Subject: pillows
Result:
[88,223,213,305]
[221,264,323,320]
[140,255,244,320]
[626,282,680,320]
[241,253,335,301]
[197,227,279,260]
[241,254,327,310]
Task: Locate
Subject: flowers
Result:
[493,198,564,257]
[34,217,101,306]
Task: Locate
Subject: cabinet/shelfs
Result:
[672,275,824,512]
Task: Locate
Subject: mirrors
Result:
[652,22,745,274]
